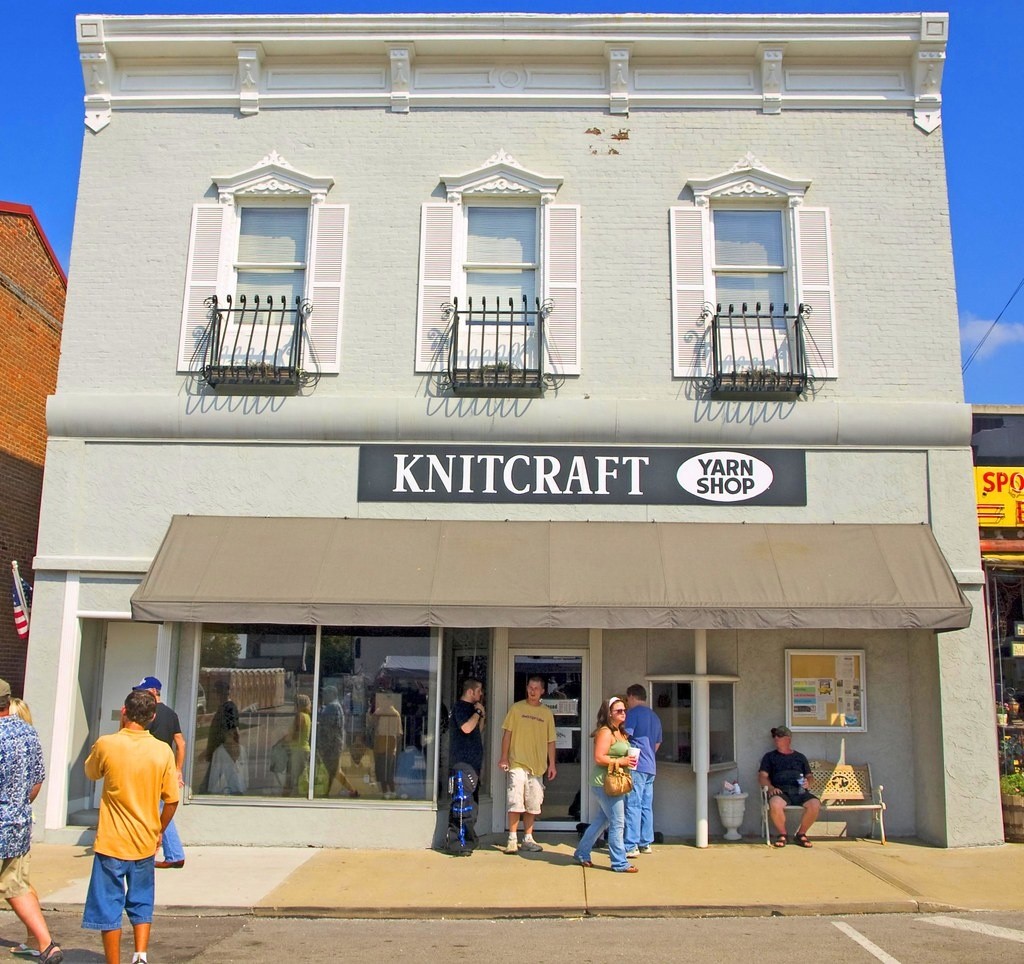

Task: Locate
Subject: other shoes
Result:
[383,791,397,800]
[582,862,593,866]
[639,845,652,853]
[625,844,640,857]
[626,866,638,872]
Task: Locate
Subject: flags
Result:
[12,559,32,638]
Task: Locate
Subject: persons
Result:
[284,695,311,798]
[317,685,359,799]
[448,678,485,842]
[758,725,821,848]
[0,679,66,964]
[498,677,556,853]
[622,684,662,857]
[367,689,403,799]
[196,679,243,794]
[10,695,42,956]
[82,689,180,964]
[134,676,186,868]
[573,696,638,873]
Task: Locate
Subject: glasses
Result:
[772,732,788,738]
[611,708,627,713]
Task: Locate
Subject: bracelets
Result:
[482,715,486,720]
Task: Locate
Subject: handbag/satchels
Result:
[604,758,633,796]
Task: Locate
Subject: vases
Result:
[1002,794,1024,843]
[996,714,1008,725]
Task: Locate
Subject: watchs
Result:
[474,707,483,715]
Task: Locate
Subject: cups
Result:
[628,747,641,770]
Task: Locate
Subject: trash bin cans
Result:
[713,793,750,840]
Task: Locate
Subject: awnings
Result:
[131,517,971,634]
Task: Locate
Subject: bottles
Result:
[797,773,806,795]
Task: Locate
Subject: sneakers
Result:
[505,838,517,853]
[521,837,543,852]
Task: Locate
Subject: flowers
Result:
[1000,773,1024,795]
[996,700,1009,714]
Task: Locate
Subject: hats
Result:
[131,676,162,691]
[0,679,11,697]
[771,726,792,736]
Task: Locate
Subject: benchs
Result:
[760,762,887,845]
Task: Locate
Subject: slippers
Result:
[10,943,41,956]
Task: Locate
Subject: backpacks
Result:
[269,743,288,773]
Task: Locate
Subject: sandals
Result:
[795,831,813,848]
[775,831,789,847]
[39,941,64,964]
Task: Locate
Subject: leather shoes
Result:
[154,859,184,868]
